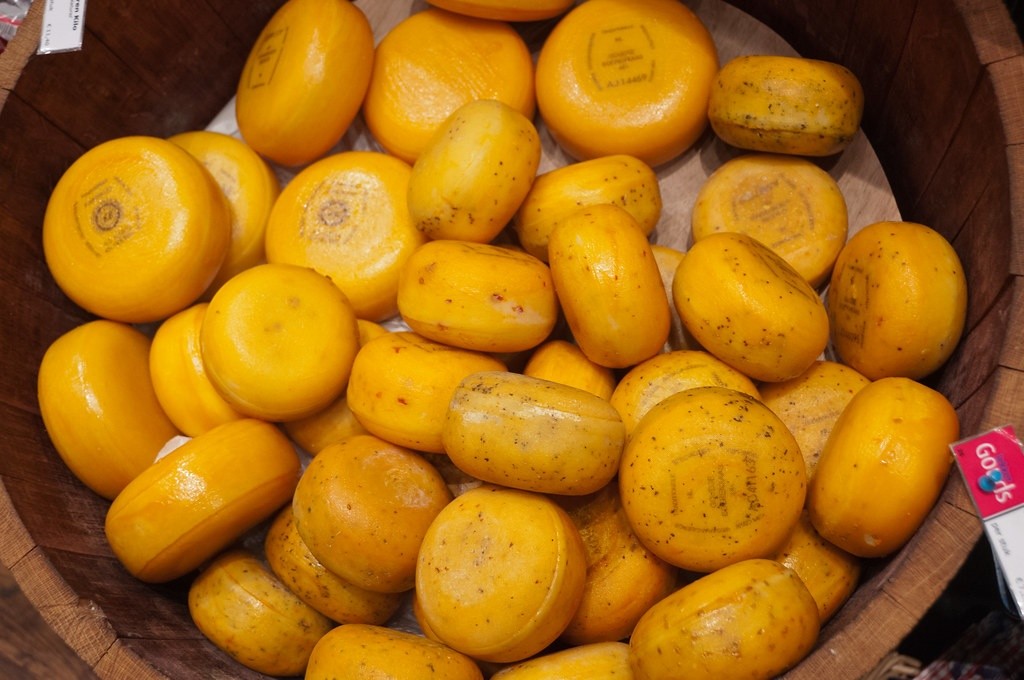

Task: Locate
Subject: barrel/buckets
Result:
[2,0,1021,680]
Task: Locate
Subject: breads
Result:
[36,0,966,680]
